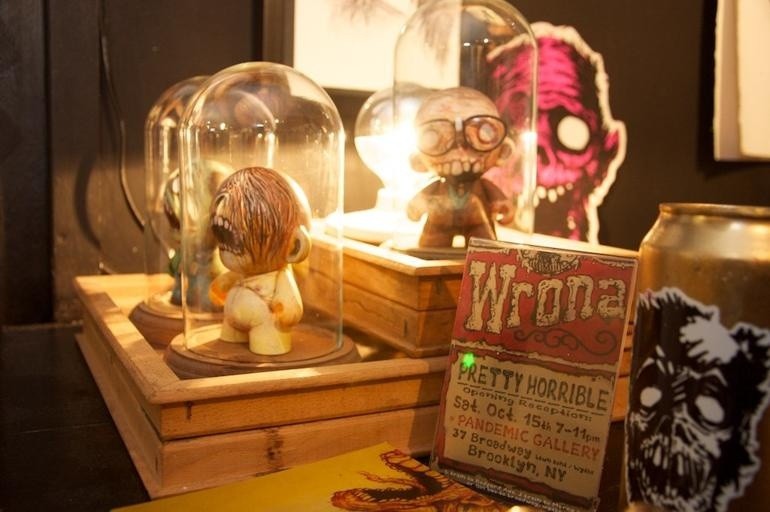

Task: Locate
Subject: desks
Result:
[0,324,625,512]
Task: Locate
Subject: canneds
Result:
[621,203,769,512]
